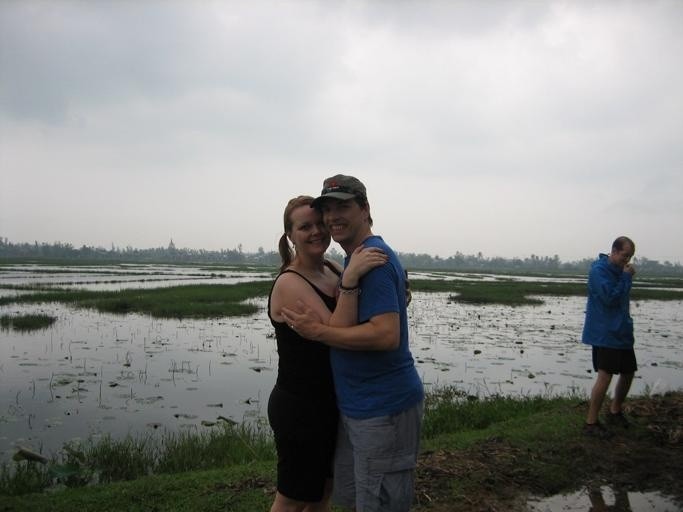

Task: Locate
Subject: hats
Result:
[310,173,367,207]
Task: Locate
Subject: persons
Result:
[279,174,425,511]
[581,235,638,438]
[265,195,388,511]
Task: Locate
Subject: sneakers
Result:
[607,407,628,428]
[584,420,604,432]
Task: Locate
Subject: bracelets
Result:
[338,281,359,294]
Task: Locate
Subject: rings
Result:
[289,323,293,329]
